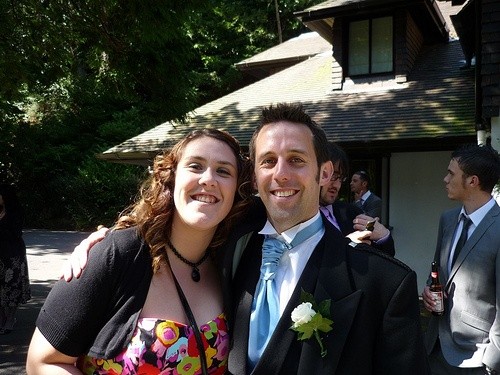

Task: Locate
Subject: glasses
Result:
[330,174,348,183]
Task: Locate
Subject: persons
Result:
[26,128,257,375]
[423,146,500,375]
[318,146,395,256]
[63,103,433,375]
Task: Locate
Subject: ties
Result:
[357,198,364,208]
[247,216,323,372]
[451,217,473,271]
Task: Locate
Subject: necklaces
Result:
[165,236,208,282]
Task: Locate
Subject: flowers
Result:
[288,288,334,361]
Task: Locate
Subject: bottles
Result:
[430,261,444,315]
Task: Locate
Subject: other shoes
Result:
[0,320,16,335]
